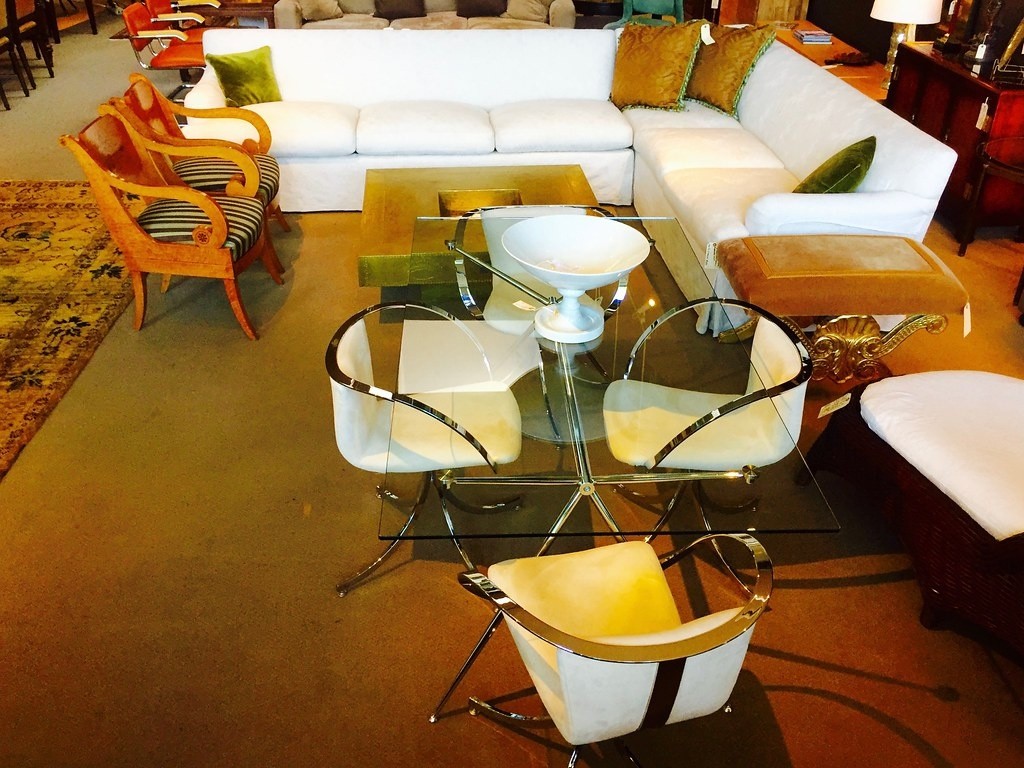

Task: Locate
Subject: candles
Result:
[971,33,989,78]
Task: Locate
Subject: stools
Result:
[957,139,1024,307]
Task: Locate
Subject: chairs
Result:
[58,103,285,342]
[337,299,531,600]
[427,533,775,768]
[96,71,292,275]
[603,0,684,28]
[0,0,102,109]
[449,203,629,386]
[603,297,816,608]
[121,1,239,82]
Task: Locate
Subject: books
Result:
[794,30,833,45]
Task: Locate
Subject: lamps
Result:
[871,0,942,44]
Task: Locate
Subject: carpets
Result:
[0,175,147,481]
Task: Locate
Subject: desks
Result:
[757,16,892,102]
[717,234,972,384]
[884,40,1024,242]
[355,160,604,323]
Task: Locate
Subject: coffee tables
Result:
[334,211,844,613]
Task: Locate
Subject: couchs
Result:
[176,27,959,334]
[273,0,576,29]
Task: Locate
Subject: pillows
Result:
[372,0,427,19]
[299,0,344,21]
[500,0,549,22]
[681,20,778,121]
[608,19,710,111]
[456,0,507,19]
[204,43,283,108]
